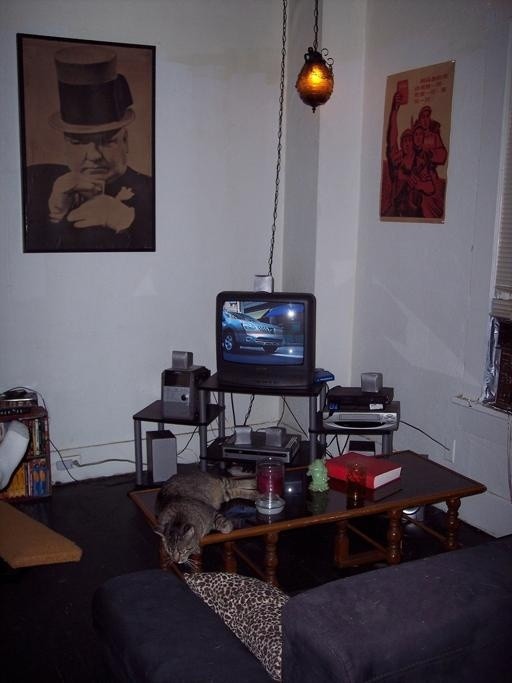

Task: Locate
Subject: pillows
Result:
[185,572,290,683]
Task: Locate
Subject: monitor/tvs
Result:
[216,291,316,390]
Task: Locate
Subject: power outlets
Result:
[62,456,81,468]
[443,439,456,462]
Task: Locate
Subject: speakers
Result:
[265,426,286,448]
[360,372,383,393]
[145,430,178,483]
[234,425,252,445]
[171,350,194,369]
[401,455,429,523]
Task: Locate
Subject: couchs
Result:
[91,533,512,683]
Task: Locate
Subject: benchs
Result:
[0,501,82,568]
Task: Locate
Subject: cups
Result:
[254,457,287,516]
[346,489,365,510]
[347,464,367,489]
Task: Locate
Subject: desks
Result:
[134,371,392,487]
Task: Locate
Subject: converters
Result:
[56,460,72,471]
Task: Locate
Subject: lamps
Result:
[295,0,335,113]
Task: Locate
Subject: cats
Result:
[151,470,261,573]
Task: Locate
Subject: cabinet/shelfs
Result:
[0,406,52,503]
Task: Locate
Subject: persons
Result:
[386,91,446,219]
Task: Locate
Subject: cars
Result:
[223,310,283,355]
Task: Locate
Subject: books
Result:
[327,451,402,490]
